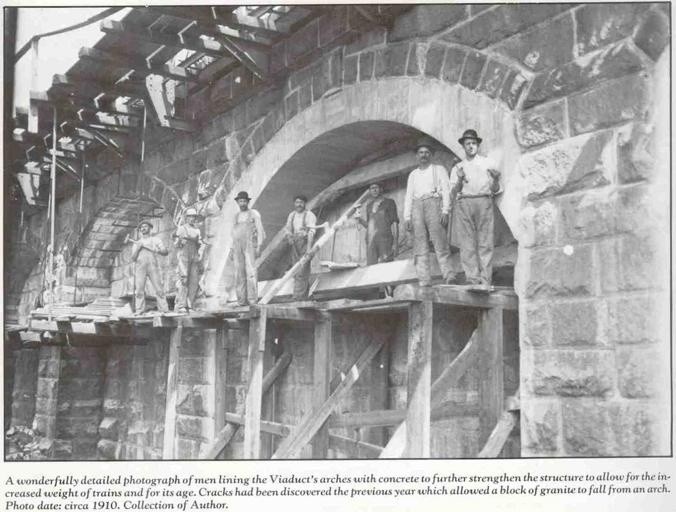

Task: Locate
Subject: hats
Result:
[458,130,482,144]
[138,219,153,228]
[186,208,197,216]
[234,192,251,200]
[415,138,432,152]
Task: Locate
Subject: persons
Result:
[404,140,458,288]
[356,182,399,299]
[283,194,317,303]
[133,221,168,316]
[230,191,265,307]
[175,208,205,312]
[448,130,505,287]
